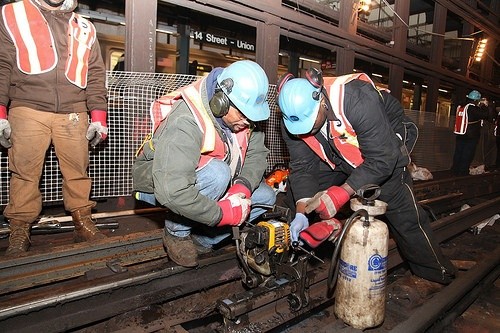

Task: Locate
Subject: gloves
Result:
[314,185,350,220]
[218,183,252,202]
[300,218,341,248]
[215,192,251,226]
[290,213,309,251]
[85,109,108,147]
[0,105,12,148]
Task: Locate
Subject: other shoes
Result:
[162,227,199,267]
[396,274,445,298]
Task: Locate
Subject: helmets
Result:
[278,77,321,134]
[468,90,481,100]
[216,60,270,121]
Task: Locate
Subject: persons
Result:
[112,53,126,95]
[493,114,500,174]
[453,89,500,176]
[0,0,109,255]
[279,71,458,291]
[189,60,198,82]
[131,59,276,267]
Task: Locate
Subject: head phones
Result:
[273,67,325,106]
[209,78,234,118]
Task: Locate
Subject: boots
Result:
[6,218,32,256]
[70,205,108,243]
